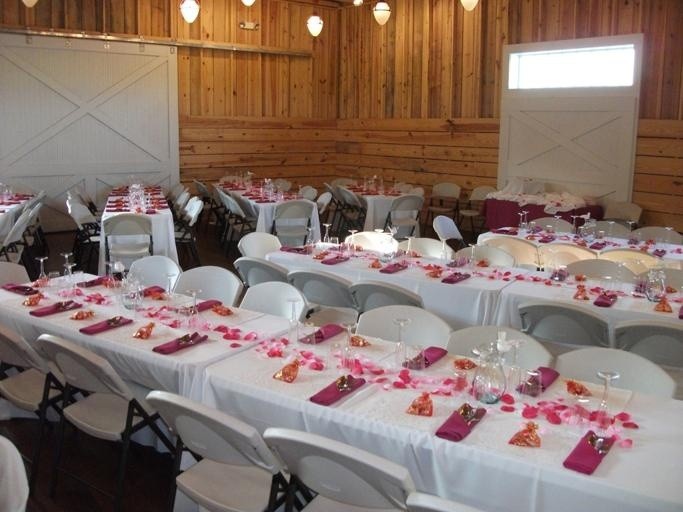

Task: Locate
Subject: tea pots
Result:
[470,342,509,406]
[644,267,667,305]
[121,269,149,312]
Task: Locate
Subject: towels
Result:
[436,404,486,442]
[402,346,447,370]
[353,190,367,192]
[223,186,239,188]
[0,210,5,213]
[150,206,169,208]
[17,194,35,197]
[146,202,166,204]
[144,192,160,194]
[530,226,542,232]
[448,259,470,268]
[29,301,82,316]
[133,286,164,299]
[590,242,606,250]
[628,238,638,245]
[275,194,292,196]
[361,193,378,195]
[594,291,616,308]
[3,283,39,296]
[229,188,246,190]
[109,194,127,197]
[2,202,20,204]
[219,184,234,186]
[248,197,268,200]
[12,198,29,200]
[321,255,348,265]
[386,193,400,196]
[112,192,129,193]
[153,333,207,354]
[106,209,129,212]
[281,246,310,254]
[653,249,665,257]
[310,375,366,406]
[300,324,342,344]
[539,236,554,243]
[179,299,221,315]
[145,186,161,189]
[79,316,132,335]
[78,276,110,288]
[144,190,161,192]
[493,228,517,235]
[112,189,127,191]
[517,367,559,396]
[241,194,259,196]
[563,431,615,476]
[148,198,166,200]
[347,188,360,190]
[679,305,683,319]
[633,282,648,297]
[256,200,275,203]
[107,205,128,207]
[284,196,303,200]
[147,210,155,214]
[108,201,128,203]
[380,263,406,274]
[550,270,569,281]
[442,272,470,284]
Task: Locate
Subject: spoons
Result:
[592,435,604,451]
[58,301,76,312]
[107,316,124,326]
[336,376,351,393]
[176,334,194,344]
[458,405,479,423]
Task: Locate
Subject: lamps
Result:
[307,1,325,38]
[460,0,480,13]
[373,2,393,26]
[182,1,200,24]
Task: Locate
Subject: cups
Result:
[375,230,384,257]
[129,182,153,213]
[600,276,612,292]
[407,346,425,379]
[363,176,388,197]
[522,370,543,403]
[232,170,287,206]
[331,238,339,245]
[301,322,319,359]
[0,183,13,205]
[565,394,592,441]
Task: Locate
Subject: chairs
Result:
[447,327,554,369]
[540,244,598,266]
[2,436,31,512]
[528,218,577,233]
[128,257,183,294]
[67,184,101,270]
[277,178,333,217]
[173,267,244,306]
[264,428,415,511]
[105,215,155,278]
[604,202,645,228]
[632,228,683,244]
[485,237,539,266]
[146,390,295,512]
[460,186,497,231]
[577,223,631,239]
[195,180,256,250]
[235,257,289,287]
[399,238,454,260]
[1,327,67,481]
[2,190,51,265]
[0,263,32,283]
[239,282,306,322]
[454,246,516,268]
[356,306,452,349]
[427,184,461,231]
[289,269,353,306]
[385,181,425,239]
[557,348,677,398]
[325,178,363,237]
[351,281,425,313]
[167,182,204,269]
[518,302,610,348]
[345,232,399,252]
[615,320,683,366]
[598,250,663,273]
[38,334,174,508]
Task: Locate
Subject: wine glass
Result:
[405,237,417,260]
[503,335,523,397]
[288,298,302,345]
[35,257,49,291]
[469,245,479,271]
[131,281,146,324]
[606,222,615,238]
[441,239,448,264]
[393,318,412,362]
[523,212,529,230]
[627,221,635,240]
[555,217,562,233]
[570,216,578,237]
[632,258,645,298]
[518,214,524,230]
[372,230,395,264]
[187,291,204,329]
[107,262,117,292]
[665,228,675,248]
[60,252,74,281]
[592,373,621,440]
[613,260,627,292]
[341,322,360,370]
[304,228,316,256]
[349,231,358,256]
[165,273,178,310]
[64,264,77,300]
[323,224,332,243]
